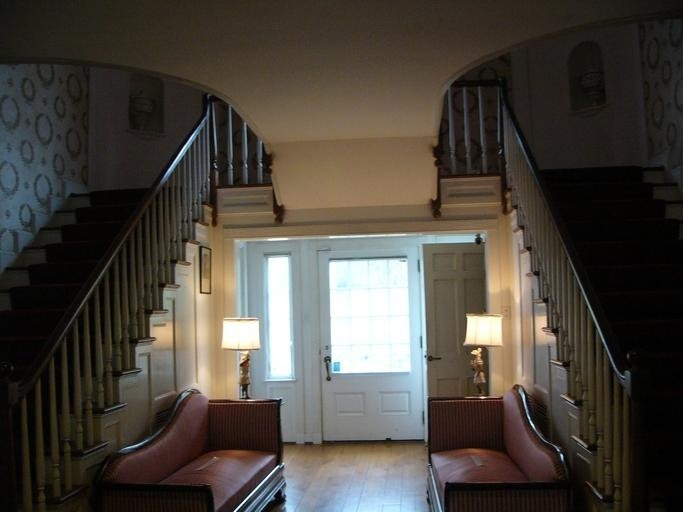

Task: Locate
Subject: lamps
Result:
[463,313,504,395]
[219,316,261,397]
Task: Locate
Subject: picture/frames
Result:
[199,245,211,294]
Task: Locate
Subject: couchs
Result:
[426,383,573,512]
[92,389,286,512]
